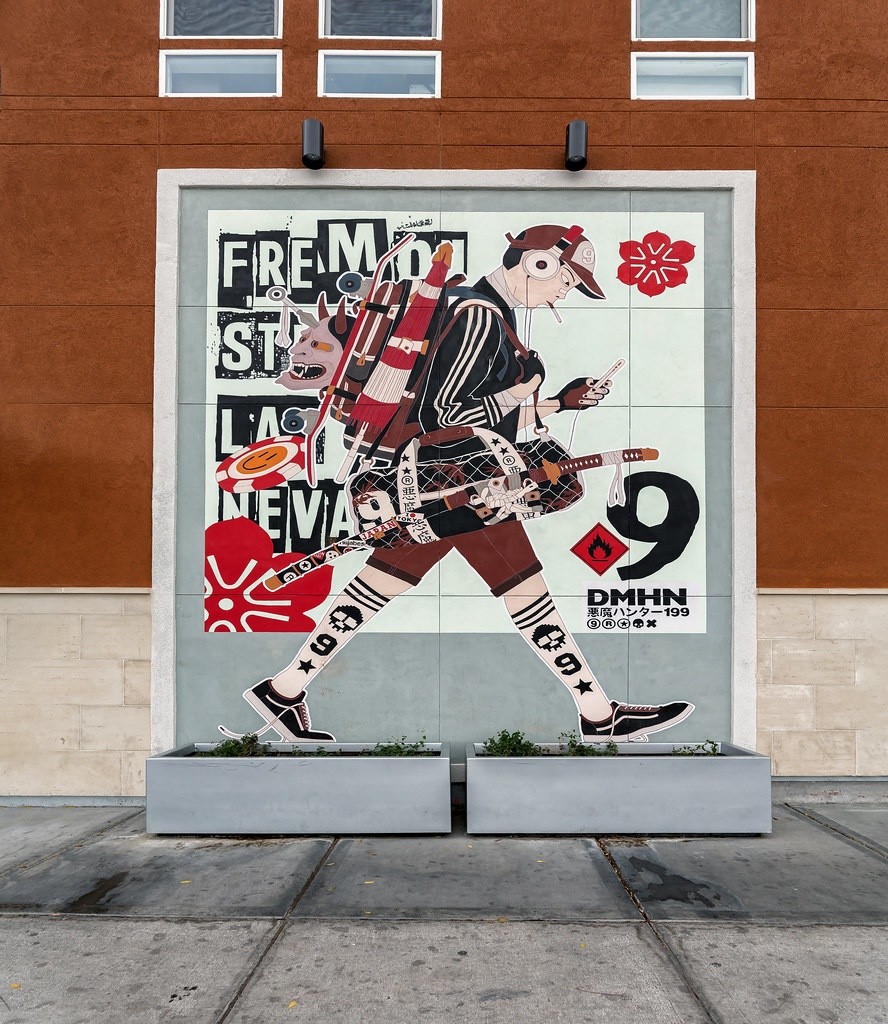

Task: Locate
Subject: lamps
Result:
[566,120,588,171]
[301,119,324,170]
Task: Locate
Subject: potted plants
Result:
[466,729,772,833]
[146,730,451,834]
